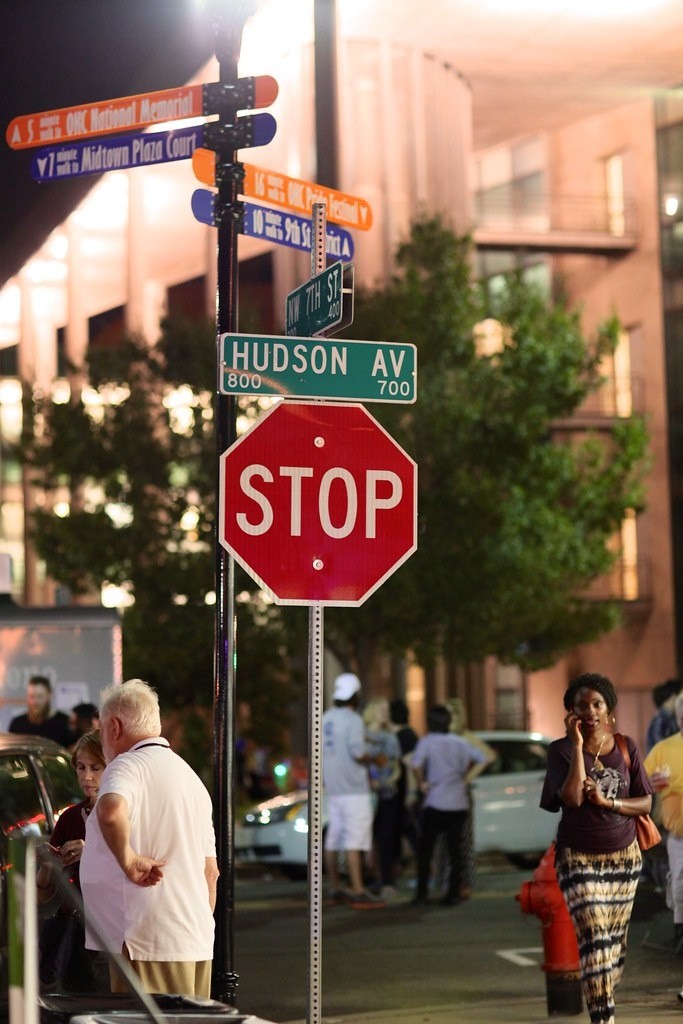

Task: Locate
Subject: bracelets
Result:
[38,884,50,889]
[609,797,622,813]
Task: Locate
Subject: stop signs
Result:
[220,402,417,603]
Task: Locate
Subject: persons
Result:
[37,678,218,1000]
[9,676,101,753]
[643,678,683,943]
[539,672,654,1024]
[322,673,496,908]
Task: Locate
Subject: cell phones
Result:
[570,712,585,734]
[38,843,61,861]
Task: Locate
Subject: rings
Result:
[71,850,75,858]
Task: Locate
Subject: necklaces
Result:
[584,732,605,772]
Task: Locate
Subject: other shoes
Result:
[347,890,389,909]
[323,889,347,905]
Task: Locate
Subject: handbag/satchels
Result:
[635,812,662,850]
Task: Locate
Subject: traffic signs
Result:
[188,146,373,259]
[7,77,281,180]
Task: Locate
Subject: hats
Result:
[331,672,362,701]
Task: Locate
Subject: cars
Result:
[235,733,565,881]
[0,730,85,1023]
